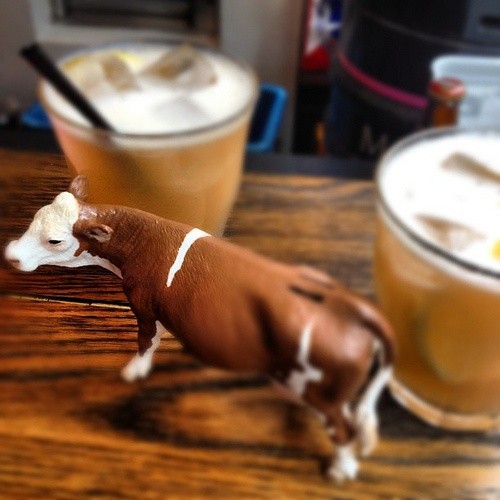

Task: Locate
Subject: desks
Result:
[0,149,500,500]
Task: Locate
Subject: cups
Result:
[371,123,500,440]
[429,54,500,125]
[38,41,259,239]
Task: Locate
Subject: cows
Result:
[4,176,400,484]
